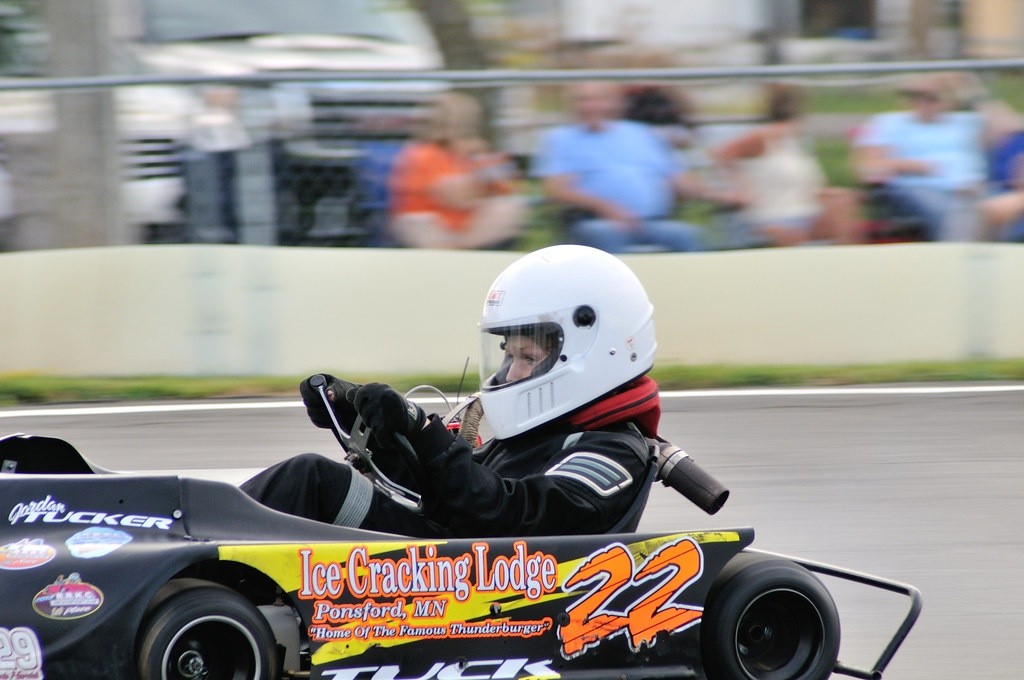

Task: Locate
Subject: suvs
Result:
[95,2,454,237]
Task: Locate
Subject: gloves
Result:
[300,376,365,428]
[346,382,426,449]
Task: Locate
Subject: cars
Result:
[2,6,248,251]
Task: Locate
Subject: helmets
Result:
[478,244,656,439]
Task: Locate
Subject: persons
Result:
[356,71,1024,254]
[240,243,661,537]
